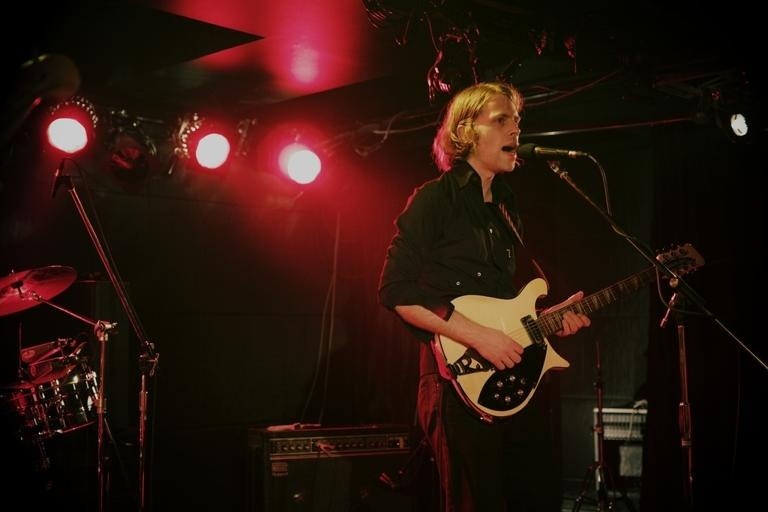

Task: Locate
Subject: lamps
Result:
[262,124,324,186]
[104,125,146,174]
[167,112,235,175]
[47,98,98,159]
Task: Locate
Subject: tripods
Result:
[572,342,638,512]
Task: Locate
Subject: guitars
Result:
[431,242,705,425]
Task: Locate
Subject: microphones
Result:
[50,160,64,198]
[515,144,589,160]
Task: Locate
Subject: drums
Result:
[0,363,110,444]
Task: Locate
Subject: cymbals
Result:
[0,264,77,316]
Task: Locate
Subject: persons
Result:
[378,81,591,512]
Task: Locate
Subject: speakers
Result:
[248,422,417,512]
